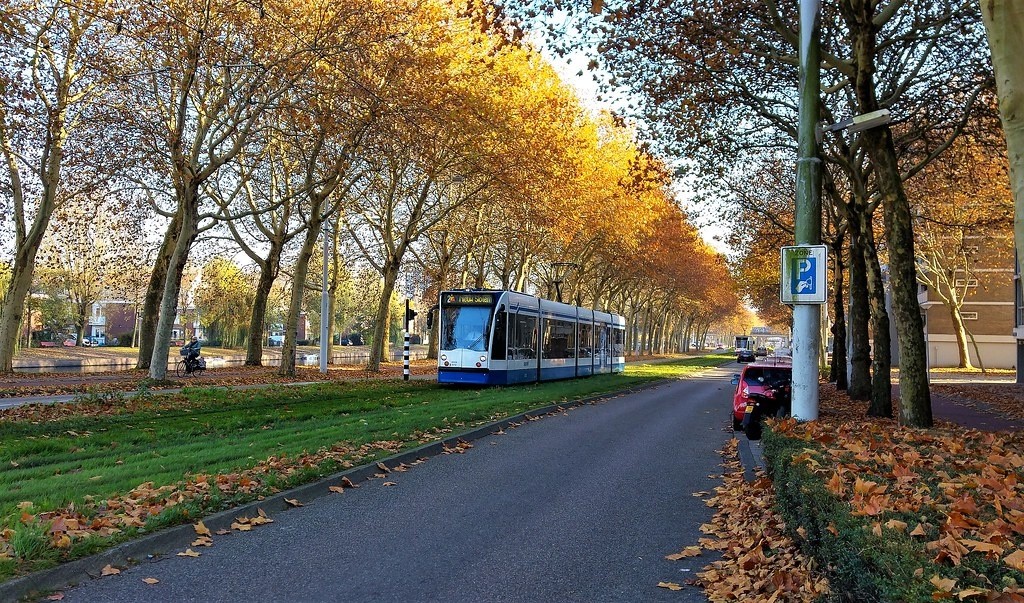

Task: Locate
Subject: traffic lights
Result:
[409,309,417,320]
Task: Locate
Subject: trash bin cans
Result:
[170,341,176,347]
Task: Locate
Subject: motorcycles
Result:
[742,370,792,440]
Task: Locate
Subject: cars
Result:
[692,341,708,347]
[737,350,754,363]
[731,349,795,432]
[717,344,724,350]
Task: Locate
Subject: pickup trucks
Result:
[54,333,90,347]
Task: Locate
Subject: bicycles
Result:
[177,347,206,378]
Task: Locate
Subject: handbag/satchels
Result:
[191,363,197,372]
[197,359,206,370]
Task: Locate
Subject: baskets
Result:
[180,349,188,355]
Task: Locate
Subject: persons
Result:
[182,336,201,374]
[763,345,766,350]
[464,327,483,340]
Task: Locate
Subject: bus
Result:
[427,263,627,384]
[734,335,755,355]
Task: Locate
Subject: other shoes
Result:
[186,371,191,374]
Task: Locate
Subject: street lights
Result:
[920,301,932,384]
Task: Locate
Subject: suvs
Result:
[756,347,767,357]
